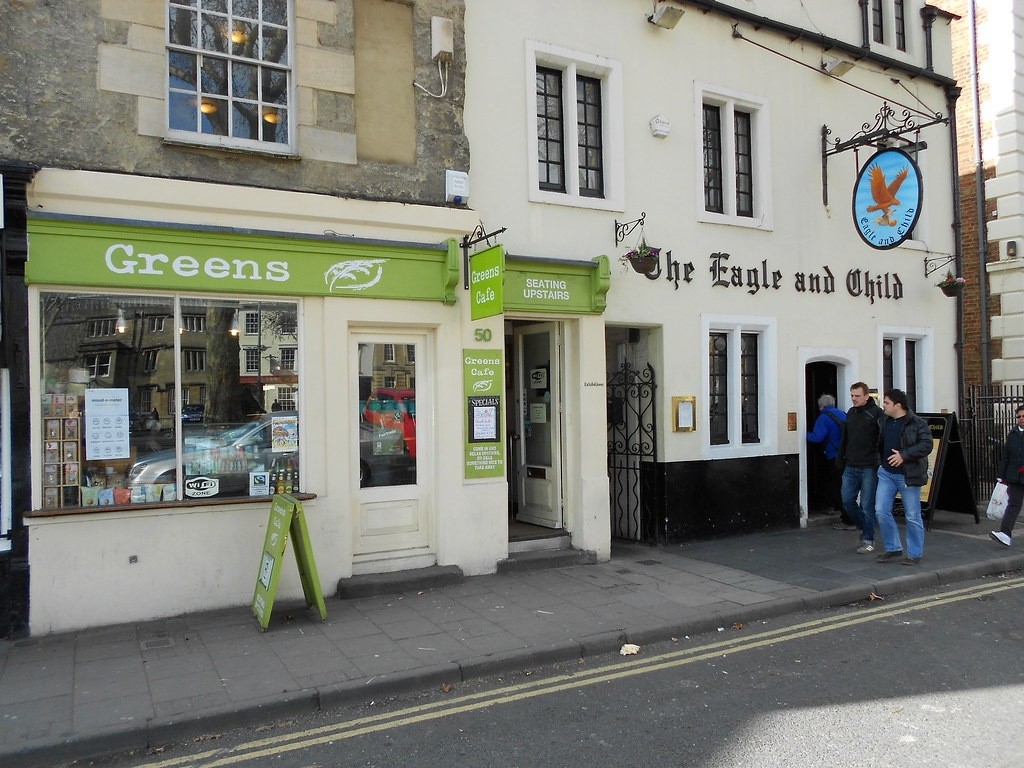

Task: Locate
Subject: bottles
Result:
[270,474,277,495]
[291,457,299,472]
[278,473,285,495]
[285,473,293,494]
[293,472,299,494]
[286,457,292,473]
[276,459,281,472]
[279,457,285,472]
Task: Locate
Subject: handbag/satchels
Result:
[986,482,1009,522]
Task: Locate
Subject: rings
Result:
[895,461,898,464]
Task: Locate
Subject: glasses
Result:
[1016,415,1024,419]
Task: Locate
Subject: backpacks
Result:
[824,409,847,481]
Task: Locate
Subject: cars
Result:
[360,388,416,458]
[125,409,407,485]
[181,404,205,424]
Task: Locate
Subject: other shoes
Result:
[831,521,857,531]
[857,543,876,553]
[819,506,835,515]
[989,531,1011,547]
[857,530,865,547]
[875,551,903,562]
[902,556,922,565]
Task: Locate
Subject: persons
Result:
[152,408,159,422]
[837,382,885,552]
[874,388,933,565]
[989,405,1024,548]
[807,395,858,531]
[272,400,281,412]
